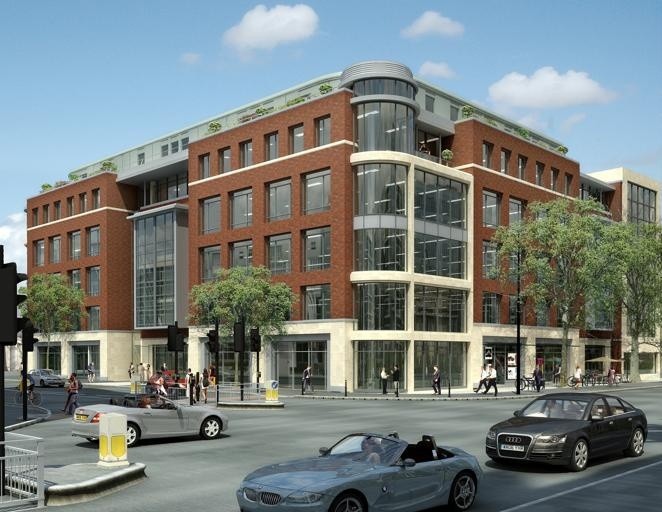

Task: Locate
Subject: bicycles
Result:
[516,372,545,392]
[15,389,44,407]
[567,369,600,388]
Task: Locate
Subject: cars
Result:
[216,366,235,382]
[28,368,67,388]
[72,392,230,446]
[162,370,189,389]
[486,393,648,470]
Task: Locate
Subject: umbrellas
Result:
[586,355,625,373]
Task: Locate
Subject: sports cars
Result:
[237,431,484,512]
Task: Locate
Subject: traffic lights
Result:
[0,262,31,344]
[207,331,219,353]
[24,323,40,351]
[251,329,261,352]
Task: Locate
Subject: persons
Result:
[481,365,498,396]
[62,372,81,414]
[608,366,615,385]
[68,376,74,415]
[390,365,400,395]
[381,367,389,394]
[574,365,581,389]
[87,360,96,382]
[431,365,442,394]
[302,366,314,392]
[21,370,36,399]
[474,366,488,393]
[553,364,561,385]
[532,364,543,392]
[128,361,215,409]
[614,373,620,384]
